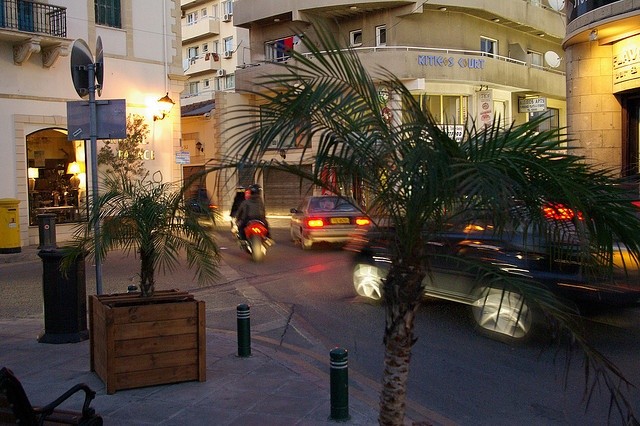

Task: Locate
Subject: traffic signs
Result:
[67,99,126,141]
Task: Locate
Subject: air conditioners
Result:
[217,69,225,76]
[222,14,230,20]
[223,50,232,57]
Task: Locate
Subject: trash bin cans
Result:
[36,213,57,249]
[38,246,90,344]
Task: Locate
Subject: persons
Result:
[233,182,272,250]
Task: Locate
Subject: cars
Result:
[290,193,373,251]
[353,223,610,346]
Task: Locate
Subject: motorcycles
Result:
[231,218,272,262]
